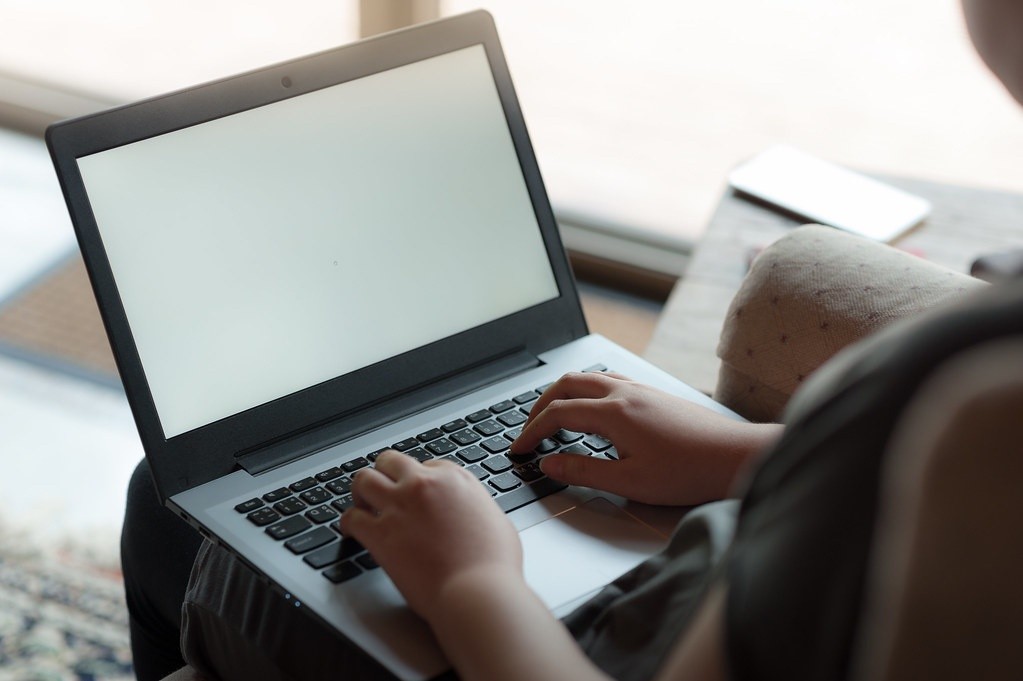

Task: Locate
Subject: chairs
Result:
[156,222,1023,681]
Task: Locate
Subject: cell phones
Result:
[729,147,931,246]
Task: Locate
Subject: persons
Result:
[120,1,1022,681]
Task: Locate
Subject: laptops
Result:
[42,9,753,681]
